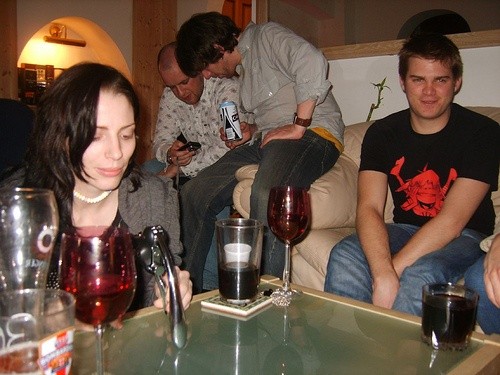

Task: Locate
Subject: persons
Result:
[173,11,346,293]
[151,42,243,291]
[0,63,192,333]
[354,308,422,375]
[257,295,334,375]
[464,233,500,338]
[72,311,192,375]
[324,33,500,316]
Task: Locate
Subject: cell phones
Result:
[177,141,201,153]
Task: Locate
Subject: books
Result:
[201,292,272,316]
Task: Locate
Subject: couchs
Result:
[233,104,500,335]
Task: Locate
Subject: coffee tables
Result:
[69,275,500,374]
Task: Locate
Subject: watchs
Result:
[293,113,312,127]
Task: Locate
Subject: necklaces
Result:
[73,190,111,204]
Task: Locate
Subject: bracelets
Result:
[167,151,172,164]
[288,318,303,328]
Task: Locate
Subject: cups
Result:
[0,184,59,291]
[215,218,264,304]
[421,282,479,351]
[0,287,76,375]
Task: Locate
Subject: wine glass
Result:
[56,223,138,375]
[267,185,311,298]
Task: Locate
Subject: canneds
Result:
[219,101,243,143]
